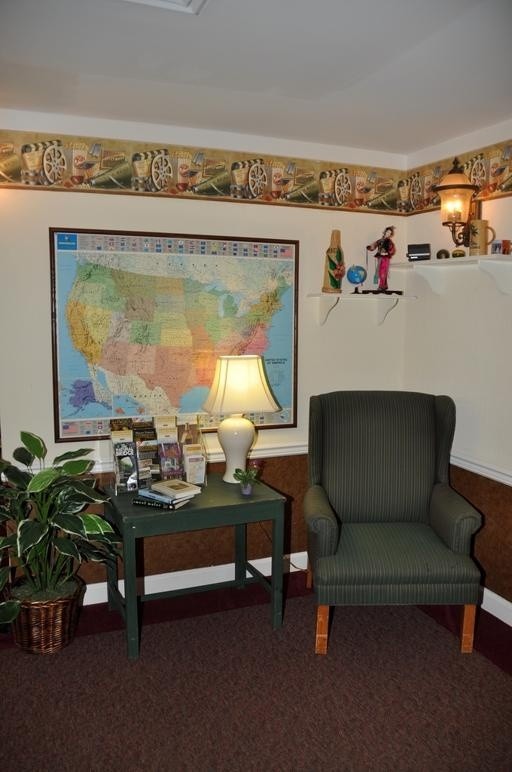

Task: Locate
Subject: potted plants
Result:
[233,467,259,496]
[0,430,125,655]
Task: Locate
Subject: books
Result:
[177,423,206,484]
[152,416,187,483]
[133,421,162,490]
[138,487,195,505]
[110,418,140,496]
[132,496,190,509]
[151,478,202,500]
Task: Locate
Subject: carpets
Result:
[1,588,510,772]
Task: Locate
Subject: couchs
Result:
[304,392,486,656]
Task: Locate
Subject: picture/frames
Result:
[48,226,300,444]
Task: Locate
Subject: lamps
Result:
[202,356,283,484]
[434,174,480,247]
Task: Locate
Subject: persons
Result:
[323,230,345,292]
[367,227,396,290]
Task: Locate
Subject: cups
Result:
[469,220,495,256]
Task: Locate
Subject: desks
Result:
[103,469,288,659]
